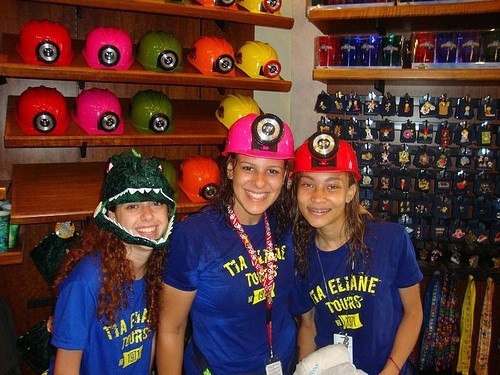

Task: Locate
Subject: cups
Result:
[0,199,19,254]
[315,27,500,66]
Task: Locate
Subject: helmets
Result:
[81,26,134,70]
[177,154,223,206]
[287,133,365,185]
[199,0,239,11]
[138,31,182,72]
[222,114,294,164]
[215,91,261,128]
[127,88,175,136]
[234,39,282,82]
[185,36,239,78]
[237,0,283,17]
[75,86,124,135]
[14,86,72,136]
[15,18,74,69]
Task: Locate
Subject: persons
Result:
[45,150,177,375]
[290,129,424,375]
[153,112,297,374]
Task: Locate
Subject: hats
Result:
[101,149,176,210]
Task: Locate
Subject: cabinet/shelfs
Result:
[304,0,500,375]
[0,0,297,375]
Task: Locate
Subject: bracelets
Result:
[389,357,401,374]
